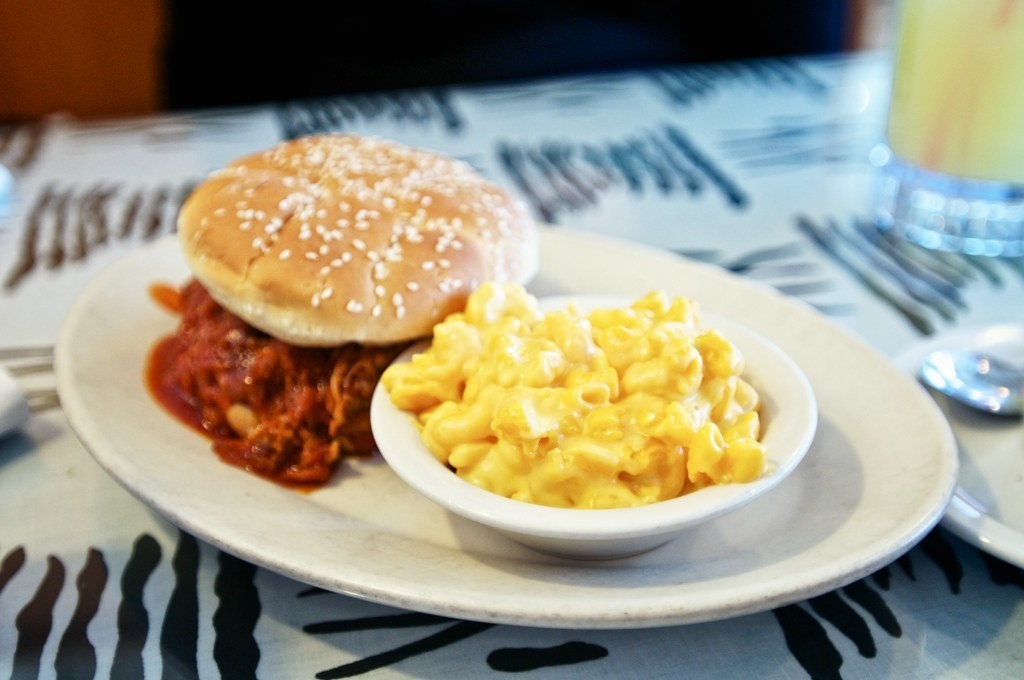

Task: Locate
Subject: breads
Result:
[176,132,538,349]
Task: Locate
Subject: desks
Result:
[0,50,1024,680]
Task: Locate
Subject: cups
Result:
[876,1,1023,257]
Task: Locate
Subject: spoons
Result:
[916,345,1024,416]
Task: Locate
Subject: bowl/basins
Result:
[370,296,818,562]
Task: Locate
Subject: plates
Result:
[894,322,1024,568]
[54,220,960,628]
